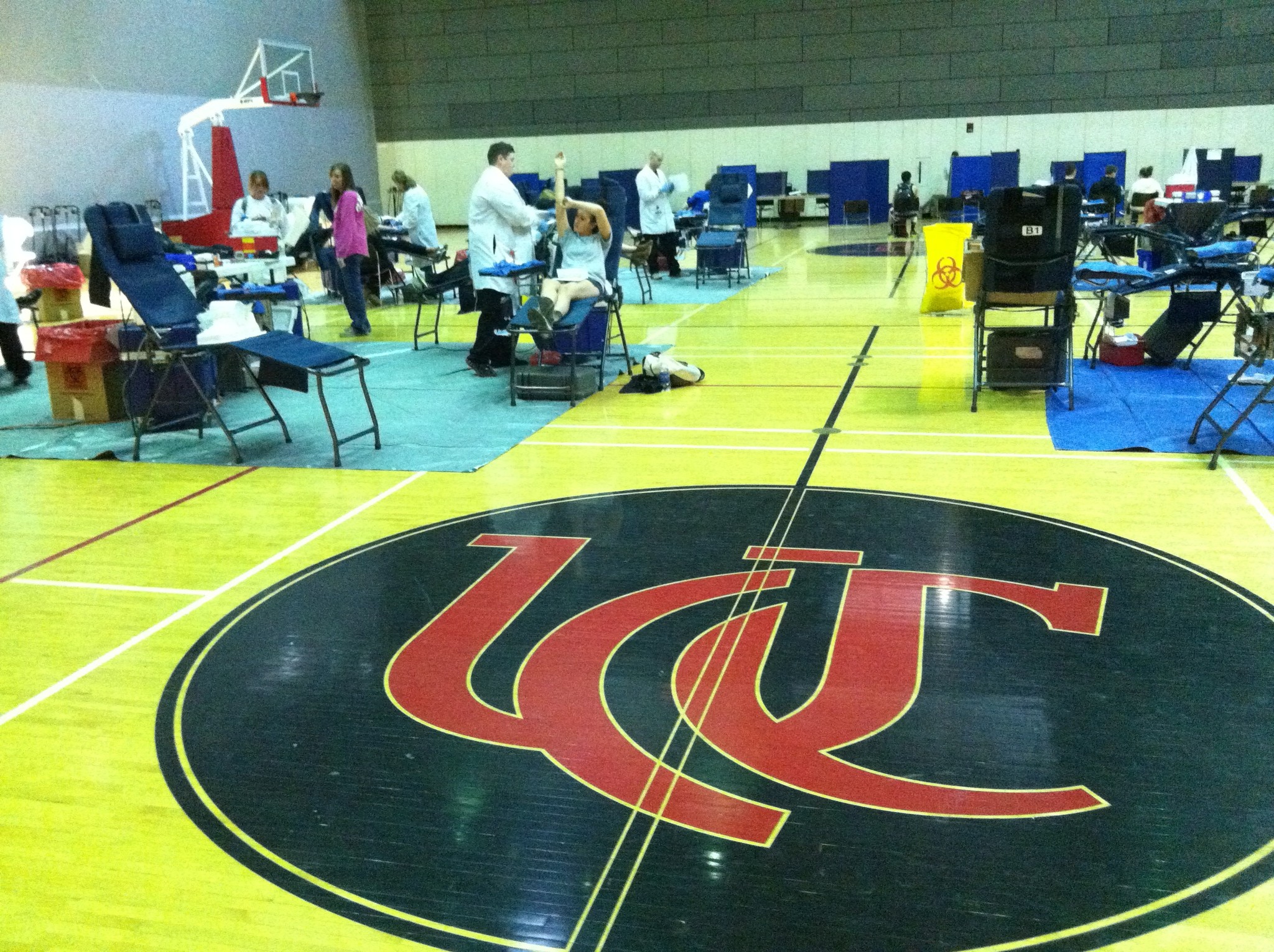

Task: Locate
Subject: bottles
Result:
[660,362,670,392]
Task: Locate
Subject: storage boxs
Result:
[227,220,279,258]
[1098,333,1147,368]
[21,262,86,323]
[33,318,138,425]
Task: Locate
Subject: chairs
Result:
[695,171,752,290]
[935,197,967,223]
[842,199,871,229]
[1075,185,1274,472]
[81,199,382,467]
[504,175,633,410]
[969,182,1083,414]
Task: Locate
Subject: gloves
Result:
[660,183,674,193]
[397,226,408,235]
[539,221,547,234]
[548,208,556,217]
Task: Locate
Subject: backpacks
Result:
[896,185,913,210]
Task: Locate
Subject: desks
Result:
[756,193,830,227]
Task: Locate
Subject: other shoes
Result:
[430,243,448,259]
[910,231,918,236]
[650,273,662,280]
[465,354,497,377]
[527,308,554,340]
[340,326,371,338]
[635,240,653,260]
[14,366,32,387]
[491,358,527,367]
[673,271,691,277]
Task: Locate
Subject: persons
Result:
[1088,165,1122,225]
[889,171,920,235]
[1127,166,1163,225]
[230,170,288,258]
[1140,199,1165,250]
[1059,165,1086,201]
[680,179,712,245]
[362,205,440,284]
[0,214,31,382]
[466,142,556,375]
[636,149,689,280]
[527,152,613,339]
[329,164,372,336]
[391,170,438,283]
